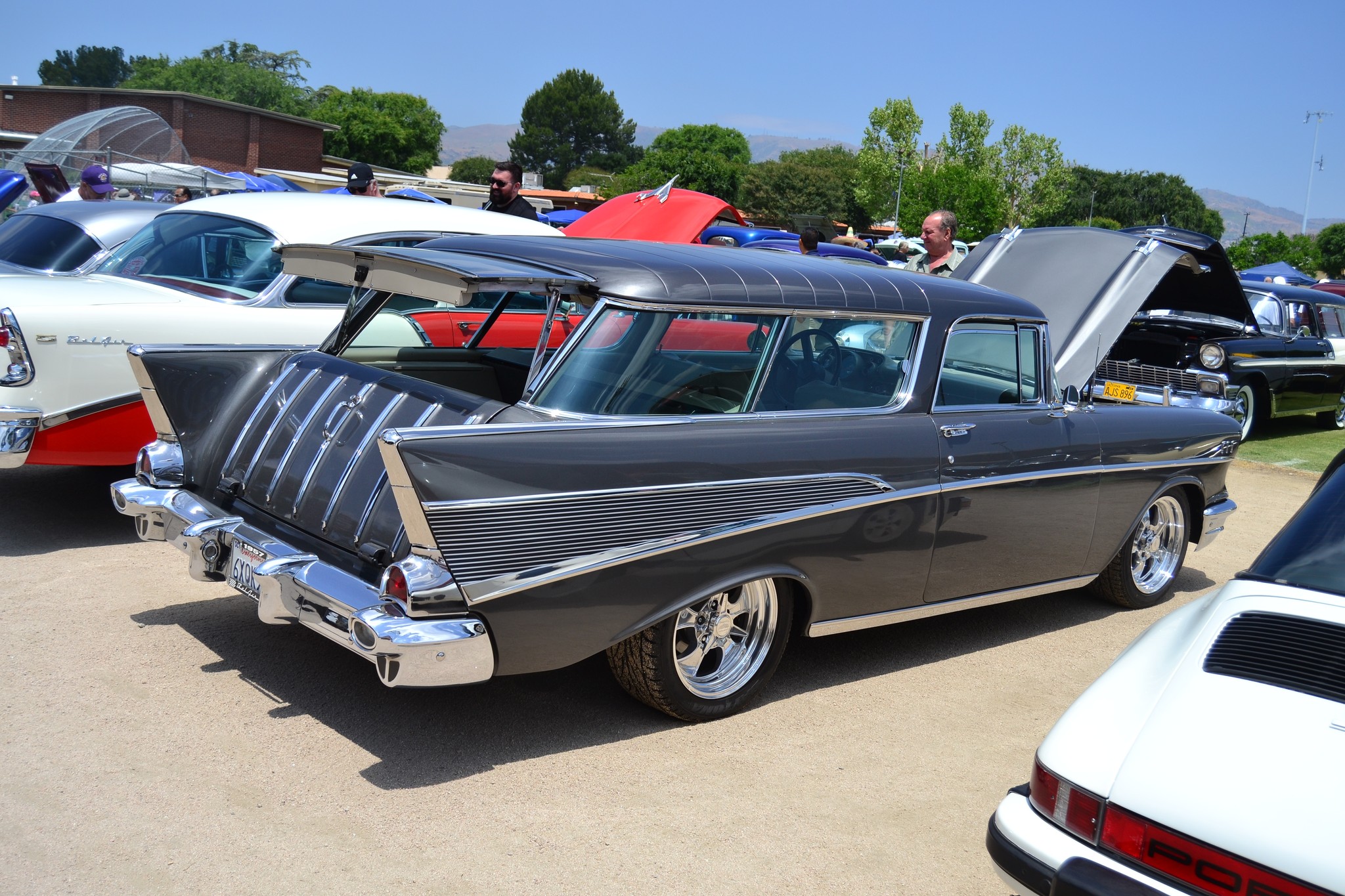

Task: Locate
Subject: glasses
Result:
[348,183,371,194]
[488,175,514,188]
[173,194,184,197]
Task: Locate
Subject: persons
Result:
[792,227,824,352]
[1297,278,1330,318]
[27,191,40,208]
[344,161,386,197]
[115,188,135,200]
[483,162,539,221]
[870,249,879,256]
[1263,276,1272,283]
[56,165,116,203]
[893,241,909,263]
[830,226,868,251]
[174,187,193,204]
[1254,276,1296,327]
[853,241,864,250]
[210,188,220,196]
[884,210,964,347]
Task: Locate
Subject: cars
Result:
[701,222,984,357]
[986,447,1345,895]
[1300,280,1345,334]
[0,199,176,275]
[0,173,771,469]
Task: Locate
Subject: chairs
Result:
[789,382,893,409]
[651,348,753,393]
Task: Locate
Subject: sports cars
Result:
[1095,226,1345,443]
[110,227,1243,731]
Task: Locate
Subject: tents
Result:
[383,188,449,205]
[1238,261,1318,288]
[320,186,352,195]
[102,162,309,197]
[544,209,588,224]
[478,207,551,227]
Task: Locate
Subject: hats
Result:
[81,165,115,194]
[115,188,136,201]
[344,163,373,190]
[133,187,142,196]
[1272,276,1286,286]
[30,191,40,197]
